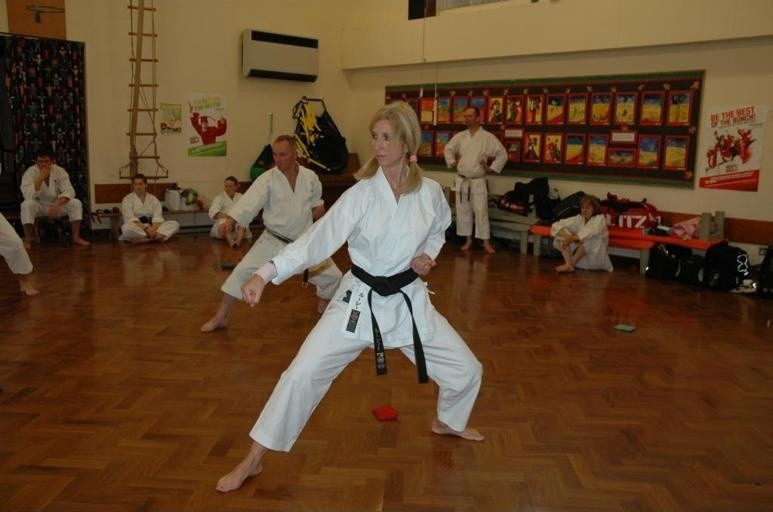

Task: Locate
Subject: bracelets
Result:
[254,262,277,284]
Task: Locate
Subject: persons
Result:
[199,134,343,334]
[19,145,92,250]
[207,175,253,247]
[442,104,511,258]
[0,212,40,297]
[117,172,179,246]
[215,98,490,494]
[549,193,616,277]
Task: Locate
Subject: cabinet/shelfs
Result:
[296,154,360,216]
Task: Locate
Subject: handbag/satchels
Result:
[550,191,661,230]
[643,242,701,284]
[497,191,528,216]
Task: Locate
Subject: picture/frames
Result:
[405,89,697,181]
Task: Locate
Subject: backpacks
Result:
[755,243,772,297]
[702,240,751,290]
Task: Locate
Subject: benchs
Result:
[0,210,123,248]
[160,208,208,215]
[449,202,729,280]
